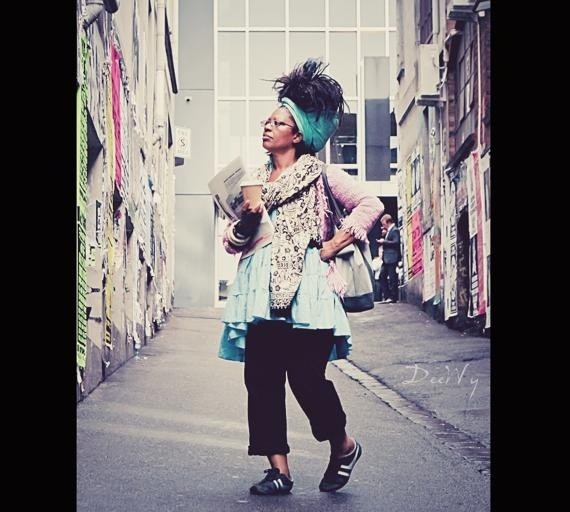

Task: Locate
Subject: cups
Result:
[239,180,264,210]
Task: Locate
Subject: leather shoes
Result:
[378,298,397,304]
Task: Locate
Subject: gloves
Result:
[233,209,262,236]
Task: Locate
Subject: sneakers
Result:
[318,437,363,494]
[248,466,296,495]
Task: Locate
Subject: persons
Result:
[215,59,387,497]
[370,213,401,303]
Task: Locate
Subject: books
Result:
[206,154,276,261]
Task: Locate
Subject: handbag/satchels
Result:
[330,233,377,314]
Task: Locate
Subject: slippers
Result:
[259,120,294,128]
